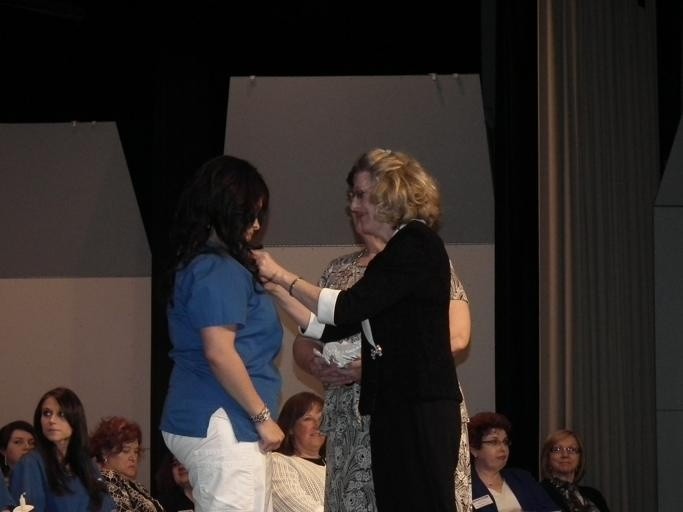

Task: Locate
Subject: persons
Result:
[268,390,328,510]
[250,151,464,511]
[0,418,38,494]
[0,469,34,512]
[465,410,562,512]
[168,456,193,512]
[538,426,611,512]
[88,415,166,512]
[290,147,474,511]
[155,153,291,511]
[9,385,119,512]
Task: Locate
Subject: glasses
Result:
[479,439,512,447]
[347,188,375,200]
[551,447,579,455]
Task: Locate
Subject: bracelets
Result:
[285,272,305,297]
[247,403,271,426]
